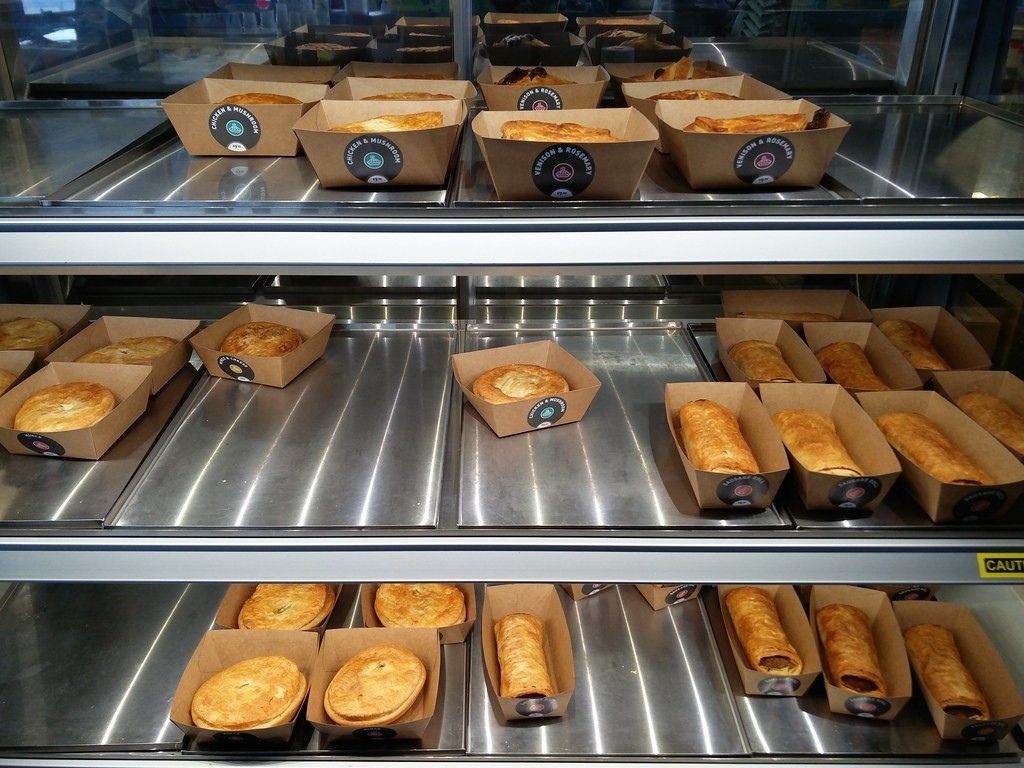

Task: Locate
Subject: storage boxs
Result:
[809,583,912,721]
[450,340,600,439]
[264,10,694,63]
[163,65,853,204]
[893,600,1024,739]
[169,630,318,743]
[663,290,1024,524]
[214,583,344,634]
[306,628,440,739]
[0,305,91,358]
[481,583,575,722]
[0,0,1024,768]
[0,350,36,398]
[0,363,153,460]
[191,304,336,389]
[717,583,821,698]
[44,315,202,395]
[360,583,477,644]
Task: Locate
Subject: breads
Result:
[730,339,798,382]
[206,68,831,142]
[904,623,990,721]
[14,381,114,431]
[879,318,950,371]
[678,398,758,474]
[238,584,335,630]
[70,335,178,365]
[472,363,570,404]
[726,586,803,675]
[956,391,1024,459]
[0,368,16,394]
[288,17,692,57]
[772,408,864,477]
[813,341,888,390]
[735,309,840,322]
[220,321,302,357]
[324,642,426,727]
[816,603,887,698]
[190,654,307,730]
[876,410,993,485]
[373,583,466,628]
[493,612,553,699]
[0,316,60,350]
